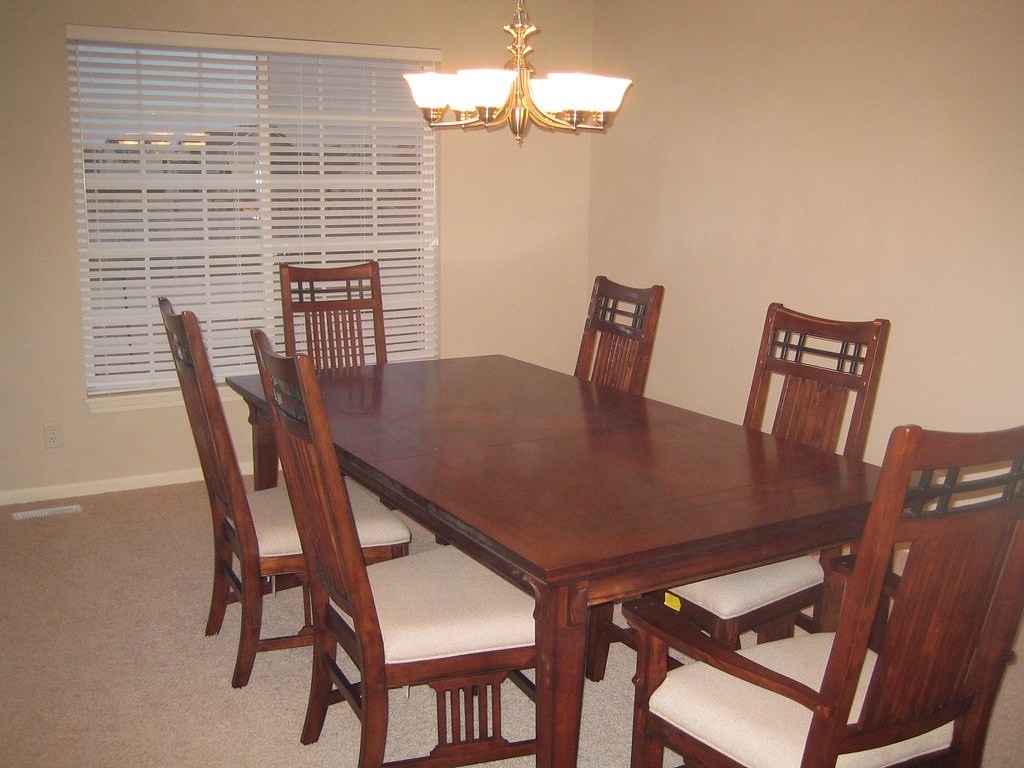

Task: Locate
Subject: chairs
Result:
[158,296,411,692]
[281,259,398,515]
[244,326,543,768]
[618,421,1024,768]
[571,273,664,398]
[584,305,893,685]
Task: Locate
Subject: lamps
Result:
[402,0,632,143]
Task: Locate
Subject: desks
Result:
[218,352,947,768]
